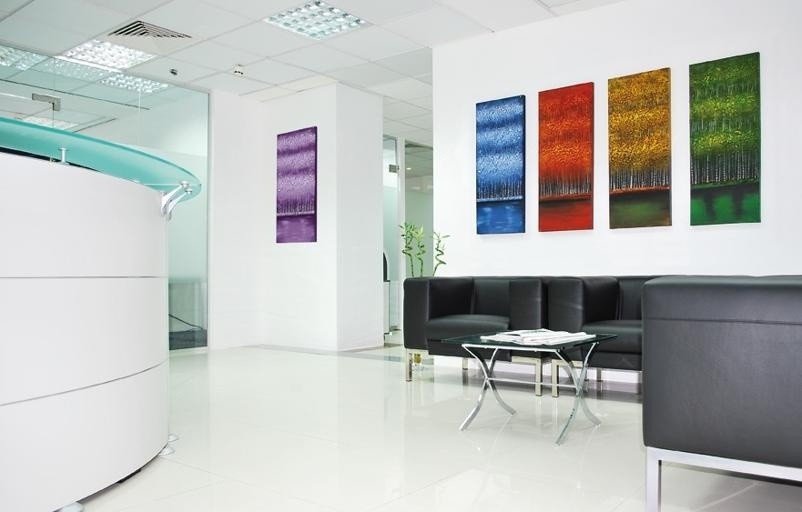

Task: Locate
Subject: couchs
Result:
[547,276,663,397]
[643,276,801,510]
[404,275,548,396]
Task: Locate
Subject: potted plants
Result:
[399,223,445,363]
